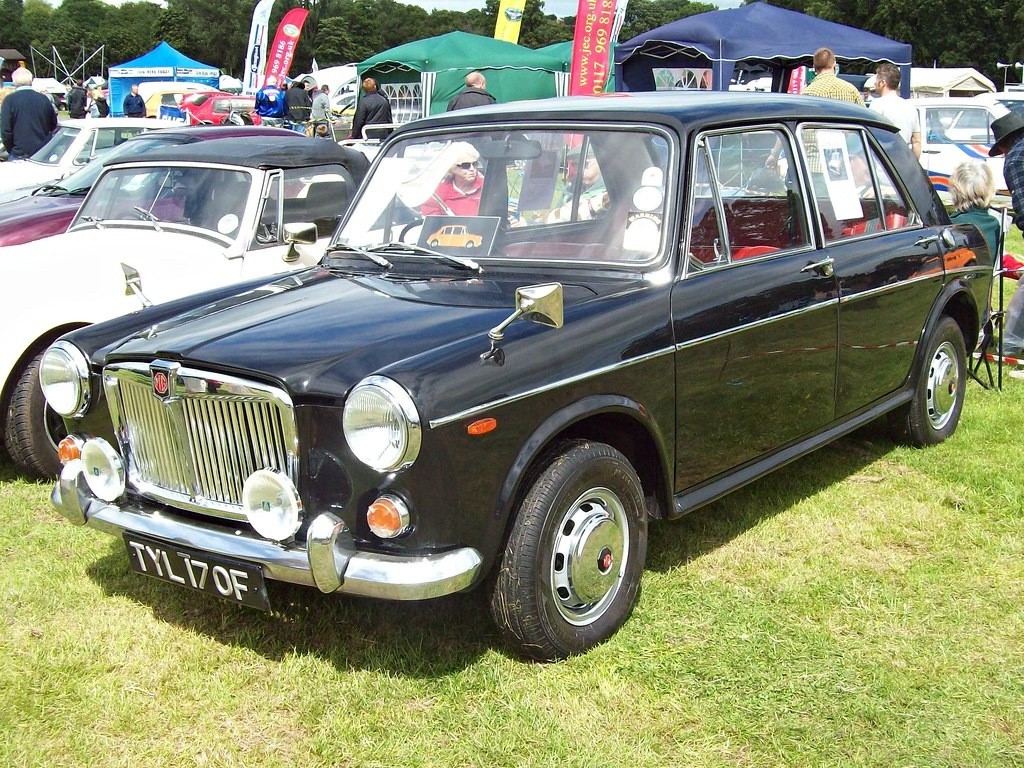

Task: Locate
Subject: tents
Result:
[864,63,998,101]
[535,35,624,95]
[613,2,912,100]
[83,75,108,89]
[354,31,563,132]
[108,39,222,119]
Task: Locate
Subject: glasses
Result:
[455,161,479,170]
[570,158,596,170]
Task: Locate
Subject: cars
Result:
[878,89,1024,194]
[0,81,439,488]
[36,92,1001,665]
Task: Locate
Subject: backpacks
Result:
[93,97,110,117]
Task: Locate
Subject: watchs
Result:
[770,149,778,158]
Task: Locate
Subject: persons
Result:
[952,157,1000,347]
[764,44,868,198]
[860,88,875,109]
[122,83,147,120]
[446,71,497,112]
[66,78,111,118]
[349,77,395,140]
[316,124,333,142]
[0,66,57,163]
[417,142,519,228]
[861,57,924,165]
[309,84,336,138]
[984,111,1024,360]
[555,143,605,212]
[254,74,319,135]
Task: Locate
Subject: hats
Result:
[565,143,596,157]
[988,110,1024,157]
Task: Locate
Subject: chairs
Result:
[504,196,905,263]
[968,208,1013,390]
